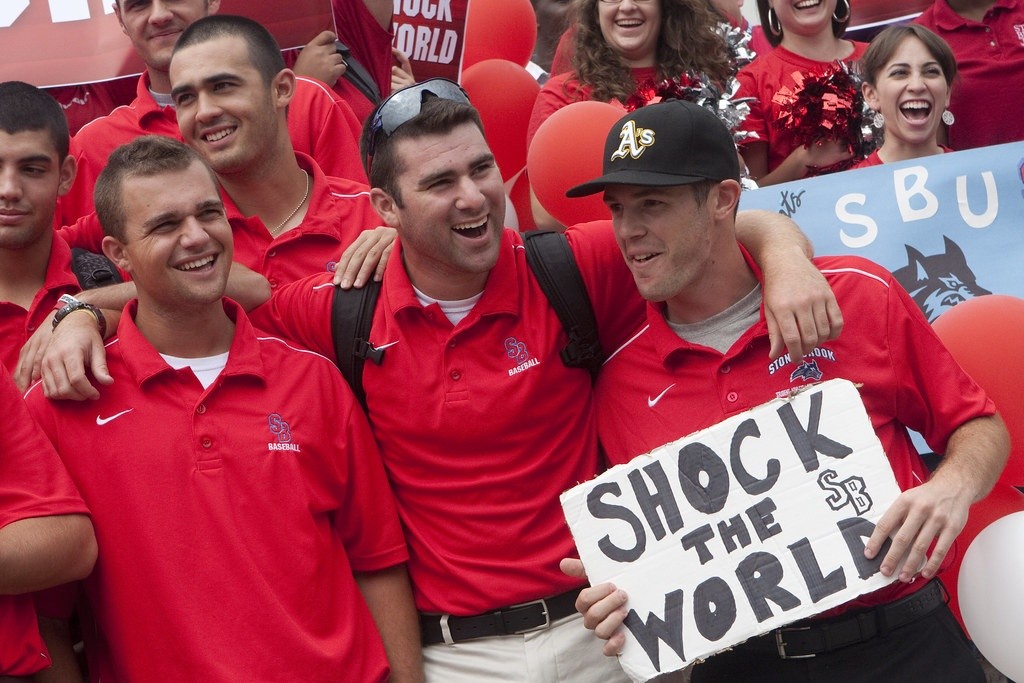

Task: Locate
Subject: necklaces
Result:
[270,169,308,233]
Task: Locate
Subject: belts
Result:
[736,579,944,658]
[417,583,591,648]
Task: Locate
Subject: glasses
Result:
[601,0,652,3]
[367,77,471,174]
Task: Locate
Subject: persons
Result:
[22,136,424,683]
[2,362,100,682]
[0,79,273,401]
[718,1,885,186]
[57,15,399,313]
[283,1,417,126]
[845,24,957,170]
[564,101,1012,683]
[52,1,371,221]
[41,77,844,683]
[910,0,1024,149]
[528,0,760,232]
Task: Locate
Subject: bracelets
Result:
[52,301,107,339]
[53,294,80,310]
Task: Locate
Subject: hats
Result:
[566,99,740,198]
